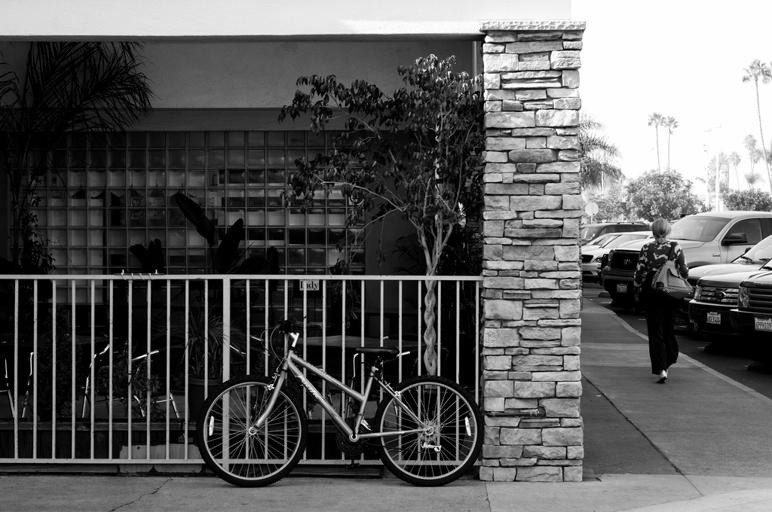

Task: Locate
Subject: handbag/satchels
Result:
[651,241,694,300]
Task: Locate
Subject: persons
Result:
[633,217,689,384]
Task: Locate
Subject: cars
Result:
[580,210,771,359]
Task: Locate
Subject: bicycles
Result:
[198,322,482,487]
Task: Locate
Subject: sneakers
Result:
[655,368,670,384]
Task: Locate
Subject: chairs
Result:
[261,318,432,432]
[1,331,182,427]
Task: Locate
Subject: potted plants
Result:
[275,51,486,478]
[151,296,257,423]
